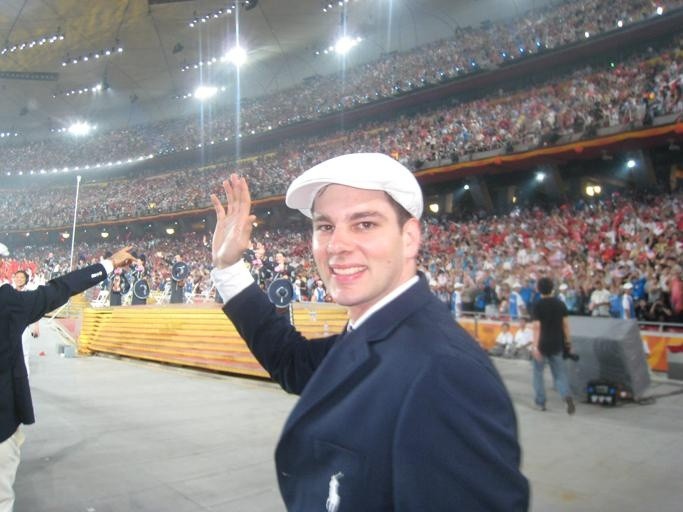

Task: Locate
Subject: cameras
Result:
[563,347,580,361]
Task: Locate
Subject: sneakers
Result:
[532,399,549,411]
[563,394,577,415]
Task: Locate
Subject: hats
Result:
[512,282,523,289]
[453,282,464,289]
[559,282,568,291]
[285,151,425,220]
[622,282,634,290]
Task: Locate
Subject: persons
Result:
[0,244,146,510]
[2,0,682,332]
[10,269,40,378]
[208,151,531,512]
[488,317,533,358]
[528,275,575,416]
